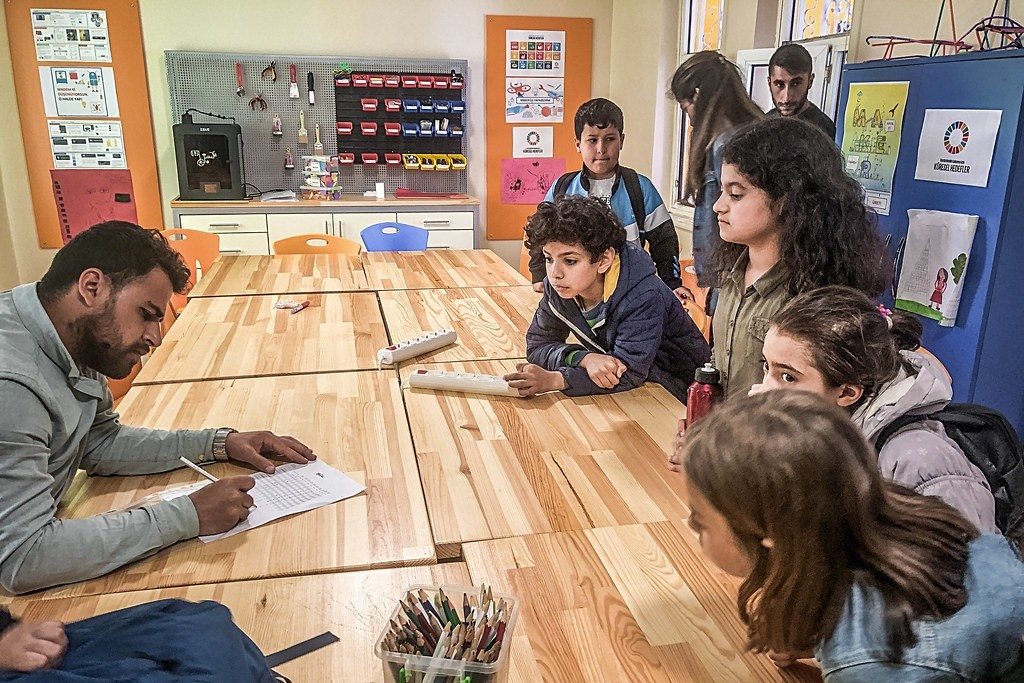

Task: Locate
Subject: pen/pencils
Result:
[380,582,512,683]
[179,456,258,508]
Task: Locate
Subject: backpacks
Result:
[875,401,1024,539]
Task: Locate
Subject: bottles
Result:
[687,363,723,428]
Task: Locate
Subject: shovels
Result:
[289,64,299,98]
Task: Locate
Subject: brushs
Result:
[313,124,324,156]
[298,109,308,144]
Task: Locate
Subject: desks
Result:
[0,248,824,683]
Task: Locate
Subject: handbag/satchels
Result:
[0,599,293,683]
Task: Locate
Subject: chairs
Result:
[272,234,361,254]
[360,220,429,251]
[151,228,221,313]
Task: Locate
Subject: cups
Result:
[376,183,384,198]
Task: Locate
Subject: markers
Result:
[291,301,310,314]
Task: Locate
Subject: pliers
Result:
[261,61,277,84]
[248,92,268,111]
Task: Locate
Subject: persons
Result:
[765,43,837,140]
[503,193,712,405]
[0,220,317,597]
[678,390,1024,683]
[0,604,69,674]
[528,98,696,305]
[664,49,766,349]
[749,286,1002,534]
[667,117,895,472]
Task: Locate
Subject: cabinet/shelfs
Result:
[172,209,475,258]
[834,48,1024,461]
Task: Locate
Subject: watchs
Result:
[212,427,239,462]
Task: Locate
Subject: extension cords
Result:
[409,369,530,396]
[378,330,456,365]
